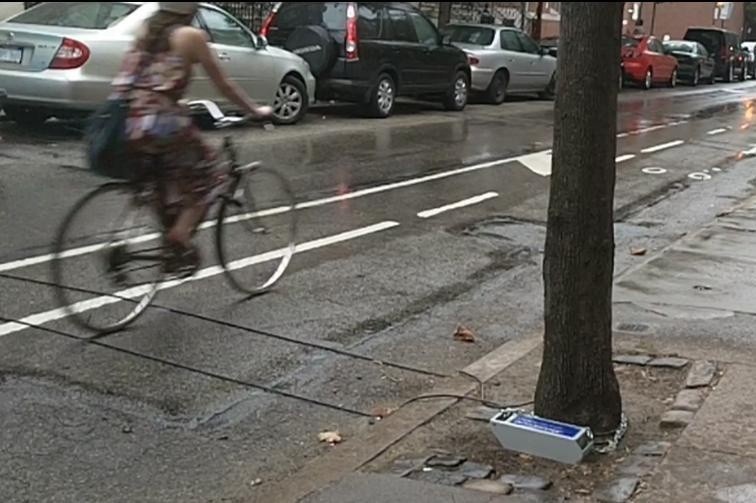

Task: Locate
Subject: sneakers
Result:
[167,228,200,264]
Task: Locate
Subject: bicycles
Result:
[54,100,298,333]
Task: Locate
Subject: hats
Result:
[158,1,193,15]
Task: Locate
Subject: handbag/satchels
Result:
[88,103,125,178]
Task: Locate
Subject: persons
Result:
[631,19,644,34]
[107,0,274,263]
